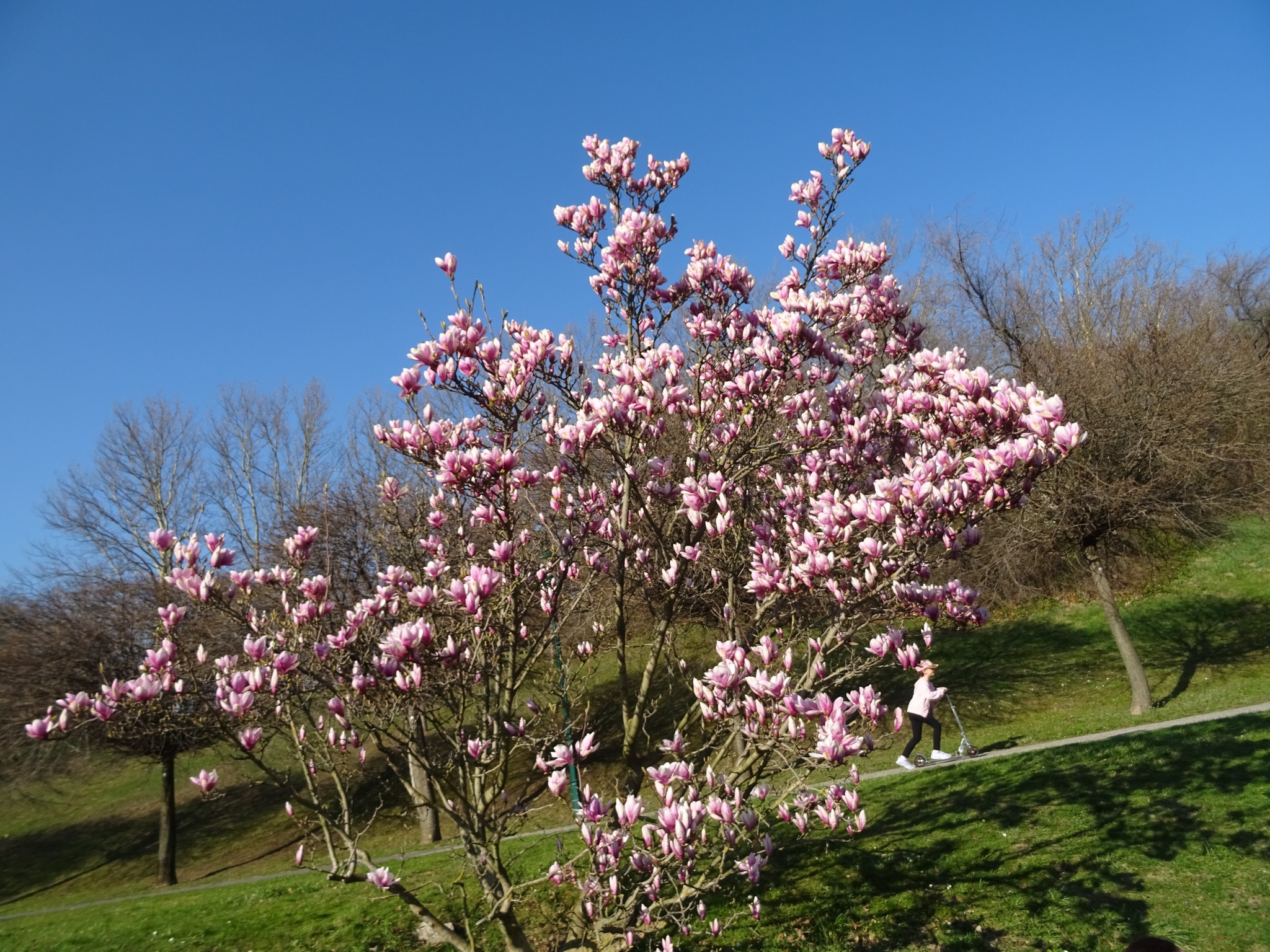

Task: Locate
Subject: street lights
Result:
[536,548,583,820]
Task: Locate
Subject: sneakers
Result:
[930,750,952,760]
[895,755,916,769]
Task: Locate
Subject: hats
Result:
[915,659,938,672]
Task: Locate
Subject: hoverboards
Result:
[914,690,980,767]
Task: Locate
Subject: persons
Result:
[895,659,952,769]
